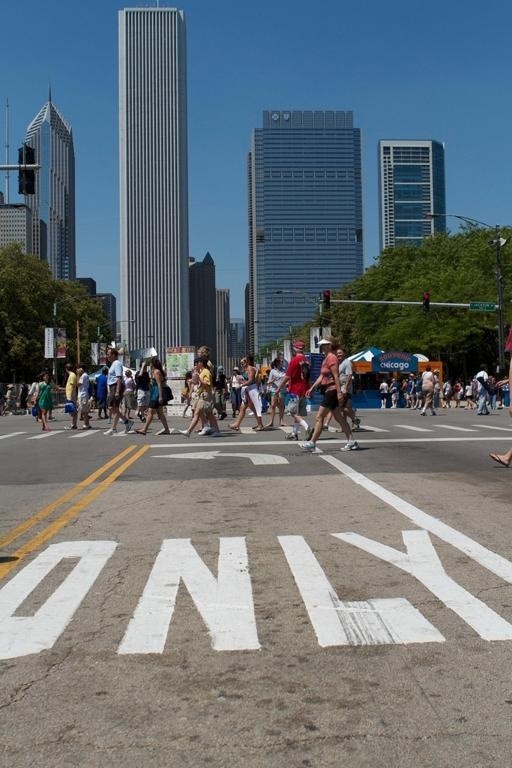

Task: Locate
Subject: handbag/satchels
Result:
[160,383,174,403]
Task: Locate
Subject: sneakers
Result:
[123,420,134,434]
[103,429,117,437]
[304,425,314,441]
[194,426,221,437]
[284,431,299,441]
[322,416,361,433]
[338,440,360,452]
[79,424,91,431]
[179,428,191,437]
[42,426,53,432]
[380,404,422,410]
[444,405,474,410]
[419,411,437,417]
[477,411,490,416]
[298,441,316,452]
[64,423,78,430]
[213,412,237,421]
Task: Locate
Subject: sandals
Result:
[251,424,265,432]
[227,421,240,430]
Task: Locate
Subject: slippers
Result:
[488,450,510,467]
[158,430,172,436]
[134,429,147,436]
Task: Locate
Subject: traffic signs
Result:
[469,302,495,312]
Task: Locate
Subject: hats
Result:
[217,365,225,372]
[232,366,240,372]
[291,339,306,350]
[316,338,333,345]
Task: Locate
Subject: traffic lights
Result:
[423,292,429,312]
[323,290,331,309]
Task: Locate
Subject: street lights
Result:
[424,212,506,380]
[53,293,110,405]
[275,289,322,354]
[97,318,137,371]
[123,333,154,366]
[248,319,293,364]
